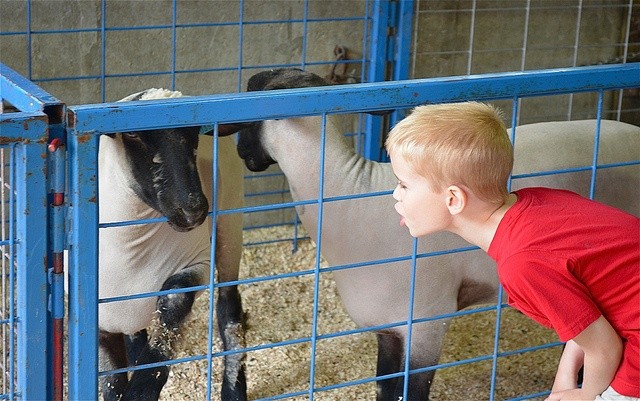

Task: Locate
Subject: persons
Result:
[383,103,640,401]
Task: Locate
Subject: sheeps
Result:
[203,62,639,401]
[55,80,260,401]
